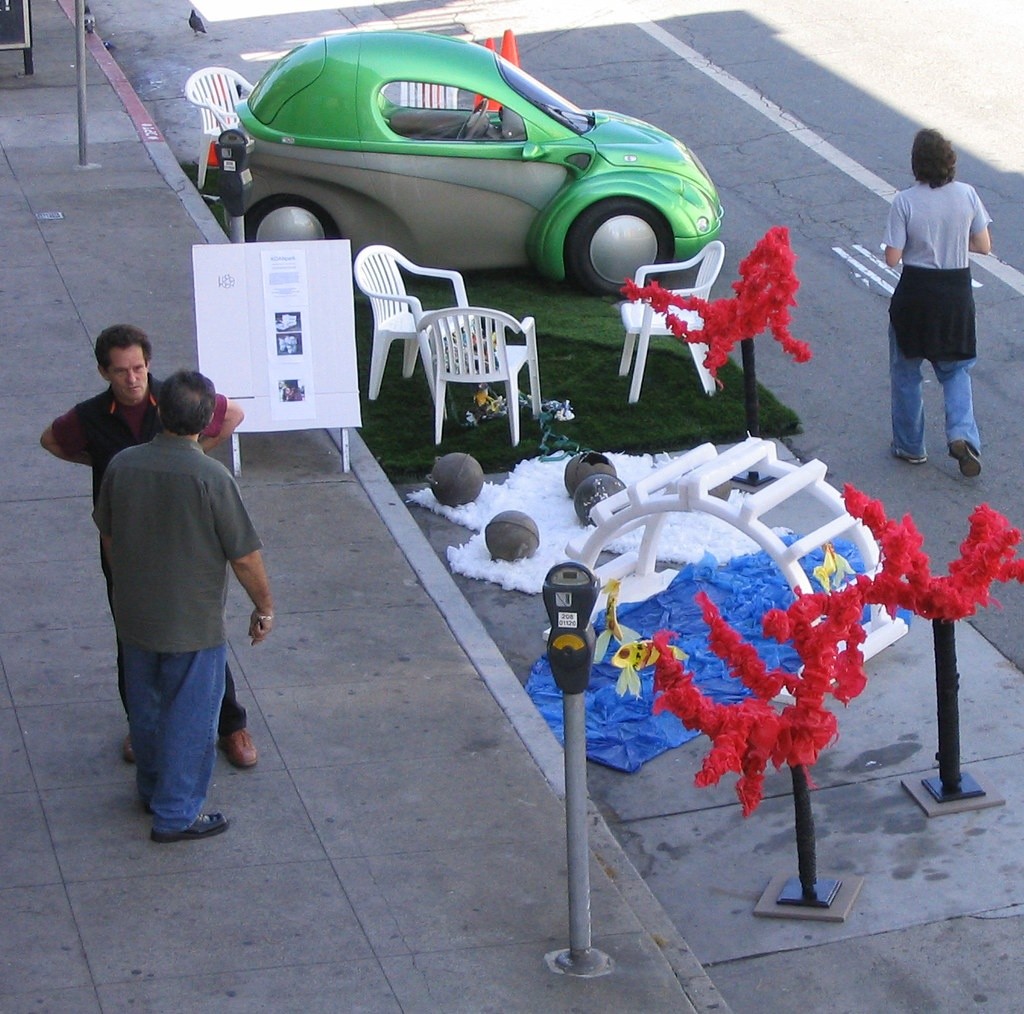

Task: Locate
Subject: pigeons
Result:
[188,10,208,37]
[84,5,95,34]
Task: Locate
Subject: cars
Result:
[224,31,725,297]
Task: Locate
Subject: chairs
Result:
[617,240,727,404]
[353,245,489,422]
[417,305,542,447]
[185,67,254,189]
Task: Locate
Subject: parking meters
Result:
[543,562,611,974]
[215,129,255,243]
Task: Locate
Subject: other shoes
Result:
[891,441,927,463]
[949,441,982,477]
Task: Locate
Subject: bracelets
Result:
[253,606,274,625]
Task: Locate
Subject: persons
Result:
[885,128,993,478]
[39,322,260,768]
[91,370,274,844]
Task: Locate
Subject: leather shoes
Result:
[219,728,256,766]
[124,733,136,762]
[151,812,229,843]
